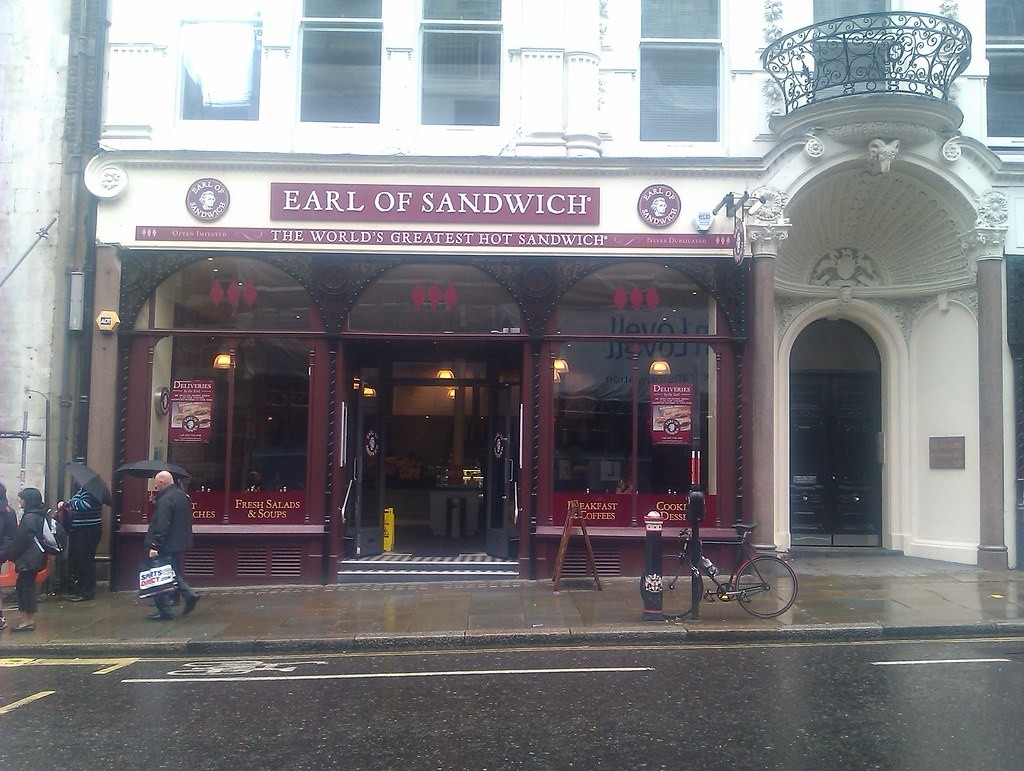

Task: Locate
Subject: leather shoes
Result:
[148,612,173,620]
[183,593,199,614]
[12,622,36,631]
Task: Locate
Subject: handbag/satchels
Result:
[139,555,179,600]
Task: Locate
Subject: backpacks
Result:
[28,508,67,555]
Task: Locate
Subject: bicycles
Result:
[639,522,799,620]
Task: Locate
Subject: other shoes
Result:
[0,615,7,630]
[150,602,157,606]
[167,600,180,606]
[67,593,93,601]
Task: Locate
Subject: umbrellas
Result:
[68,463,112,505]
[115,460,192,497]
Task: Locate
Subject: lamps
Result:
[364,387,377,398]
[648,359,672,376]
[436,368,455,379]
[553,359,570,384]
[446,387,457,400]
[212,354,237,370]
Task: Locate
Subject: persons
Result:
[0,483,17,630]
[616,477,634,494]
[144,470,200,621]
[249,443,285,491]
[8,488,47,631]
[57,485,102,602]
